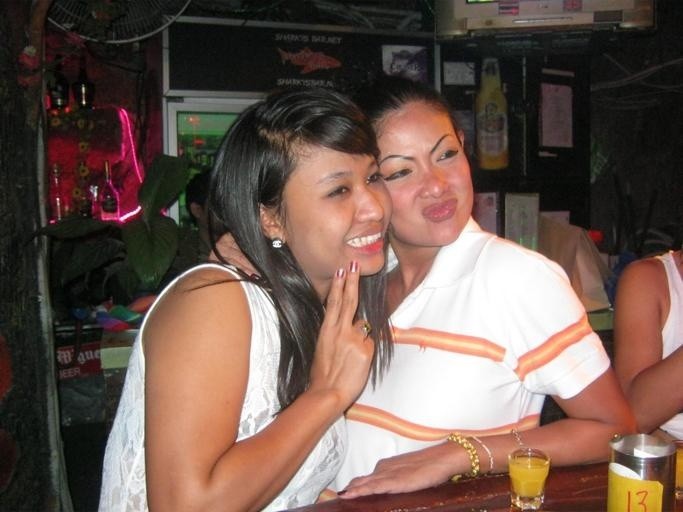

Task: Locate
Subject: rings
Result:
[359,324,369,334]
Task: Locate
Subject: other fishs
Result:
[276,46,343,76]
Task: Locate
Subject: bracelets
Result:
[444,435,480,483]
[470,434,494,471]
[509,428,525,451]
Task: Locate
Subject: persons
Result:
[93,86,401,511]
[168,168,221,271]
[609,246,682,445]
[203,83,640,501]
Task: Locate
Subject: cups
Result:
[505,446,551,510]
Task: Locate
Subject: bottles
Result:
[46,64,69,111]
[608,432,676,512]
[99,160,121,220]
[72,60,95,111]
[472,56,511,173]
[49,162,69,221]
